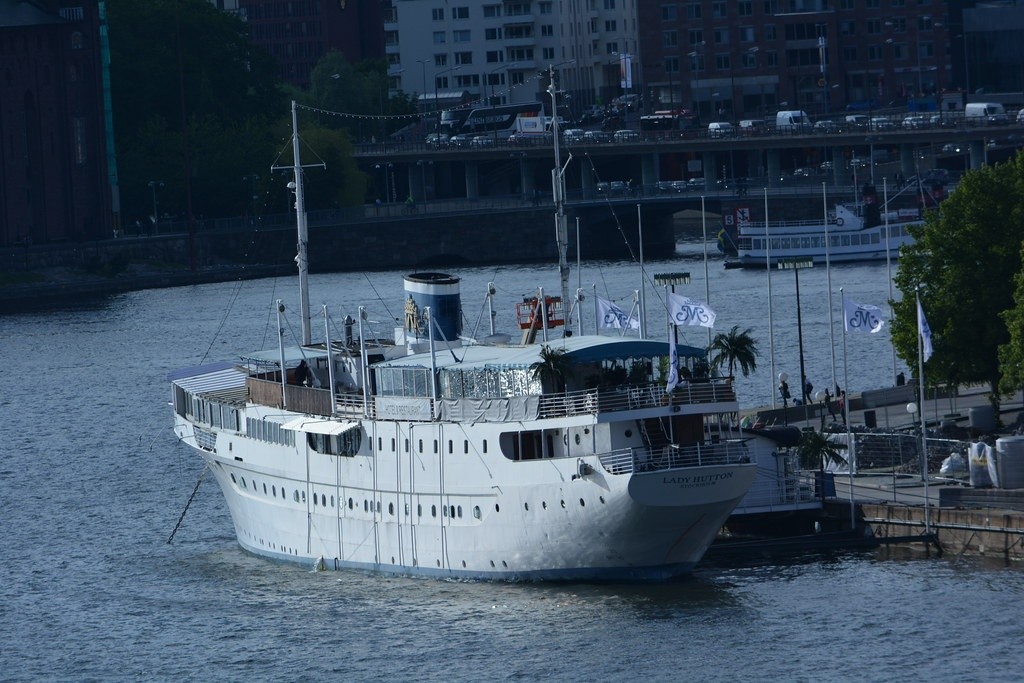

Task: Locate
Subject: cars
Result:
[564,129,638,141]
[424,134,492,149]
[816,120,838,131]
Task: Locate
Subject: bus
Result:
[440,101,547,138]
[639,110,699,141]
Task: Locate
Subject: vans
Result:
[708,122,735,137]
[776,110,811,133]
[965,103,1009,123]
[738,119,769,132]
[846,115,874,127]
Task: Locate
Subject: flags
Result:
[918,302,932,363]
[665,328,678,393]
[668,293,717,328]
[597,296,639,331]
[843,299,884,333]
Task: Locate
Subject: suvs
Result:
[508,130,552,145]
[872,117,894,130]
[903,116,930,129]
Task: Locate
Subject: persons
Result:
[804,375,813,404]
[136,210,208,237]
[893,168,906,190]
[631,366,648,408]
[840,390,846,425]
[793,398,802,406]
[295,360,307,387]
[897,372,905,386]
[778,380,788,408]
[693,359,710,378]
[836,382,840,396]
[611,366,624,410]
[824,389,836,422]
[404,194,416,209]
[681,366,692,379]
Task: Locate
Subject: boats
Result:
[724,211,932,273]
[172,96,756,592]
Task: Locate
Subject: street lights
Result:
[434,65,462,111]
[490,61,518,146]
[545,59,577,338]
[379,68,408,152]
[415,58,434,135]
